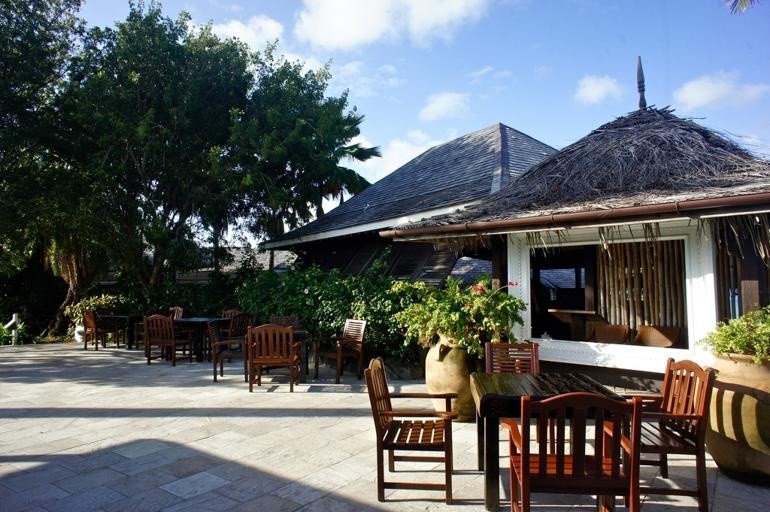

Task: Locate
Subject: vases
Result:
[423,328,486,422]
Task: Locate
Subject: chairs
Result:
[315,320,367,384]
[485,342,540,374]
[503,391,642,512]
[81,305,253,382]
[604,357,715,512]
[365,357,459,504]
[246,324,302,392]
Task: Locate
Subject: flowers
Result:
[390,271,528,359]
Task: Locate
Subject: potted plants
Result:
[693,305,770,486]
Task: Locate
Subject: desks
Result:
[469,371,627,512]
[245,329,308,382]
